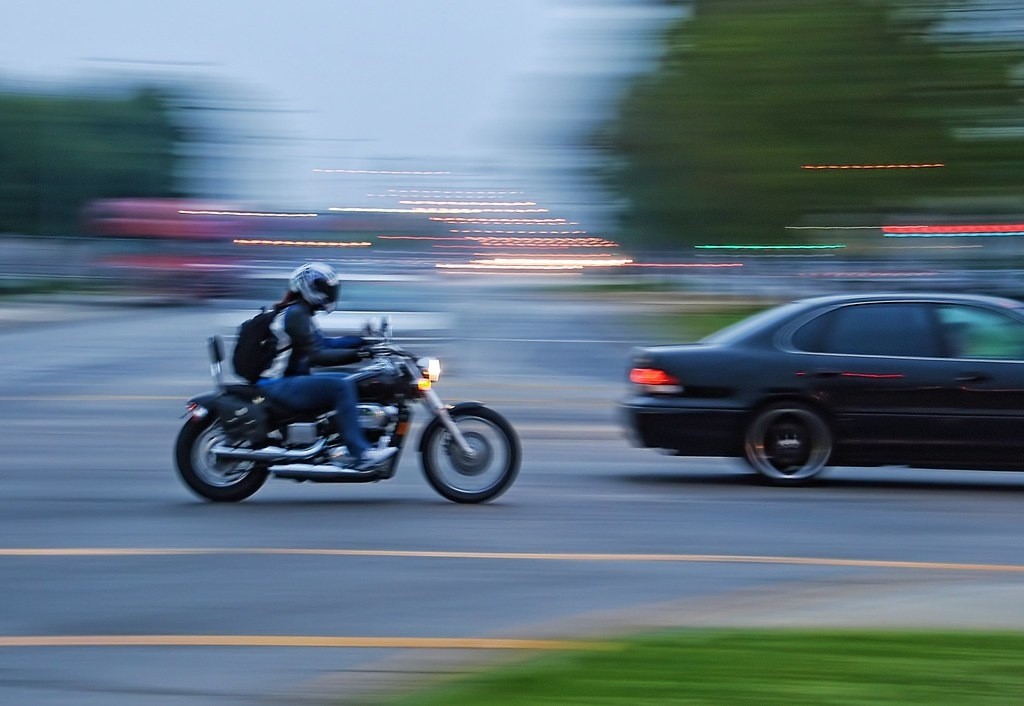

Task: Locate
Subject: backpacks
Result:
[232,299,313,383]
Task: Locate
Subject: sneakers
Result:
[355,447,399,470]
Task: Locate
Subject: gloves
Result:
[356,346,392,358]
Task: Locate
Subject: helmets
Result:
[289,262,343,314]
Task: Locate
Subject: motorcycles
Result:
[173,317,522,503]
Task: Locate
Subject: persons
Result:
[254,262,399,470]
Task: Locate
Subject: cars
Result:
[617,292,1024,485]
